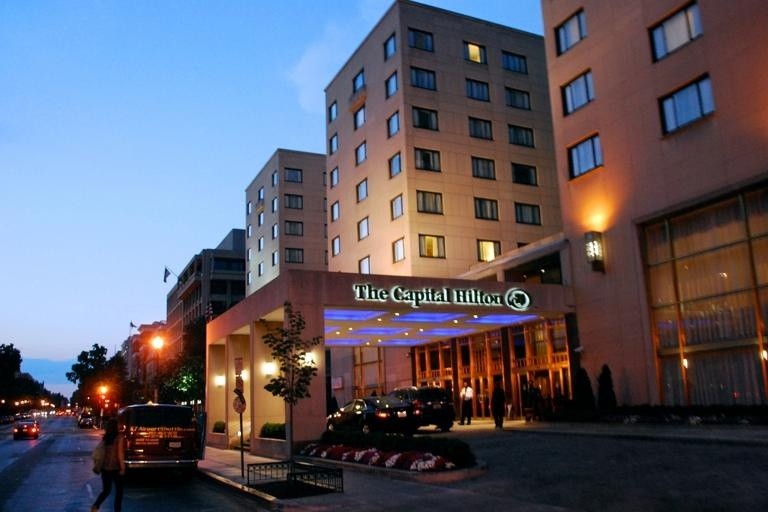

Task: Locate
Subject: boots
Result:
[90,506,98,512]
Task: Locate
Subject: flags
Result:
[163,269,171,283]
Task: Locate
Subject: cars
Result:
[76,411,94,428]
[105,402,198,485]
[14,419,41,440]
[326,386,457,436]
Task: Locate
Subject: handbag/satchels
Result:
[92,441,106,475]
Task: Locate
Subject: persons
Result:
[89,418,126,512]
[491,382,506,431]
[458,381,473,425]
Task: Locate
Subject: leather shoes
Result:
[458,422,470,425]
